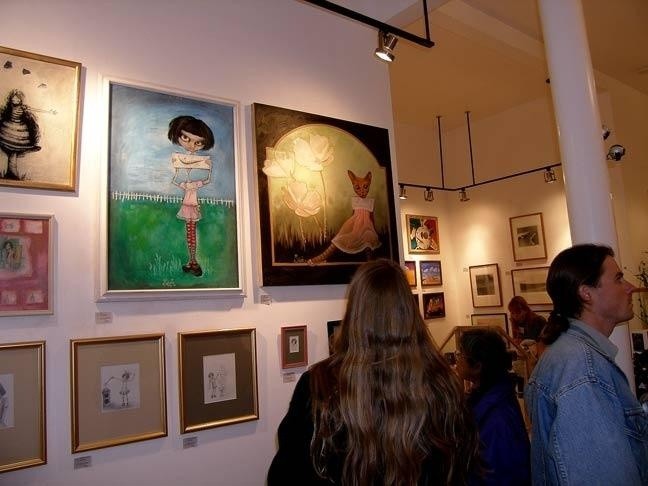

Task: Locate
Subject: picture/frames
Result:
[326,318,343,358]
[404,211,554,353]
[92,69,249,305]
[279,324,310,369]
[68,331,170,455]
[249,99,402,290]
[0,45,84,195]
[0,338,50,475]
[0,210,57,319]
[175,326,262,435]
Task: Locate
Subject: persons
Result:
[453,327,533,486]
[262,256,469,486]
[506,292,549,343]
[524,240,648,486]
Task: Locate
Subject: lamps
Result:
[374,28,398,65]
[396,165,557,203]
[602,126,626,162]
